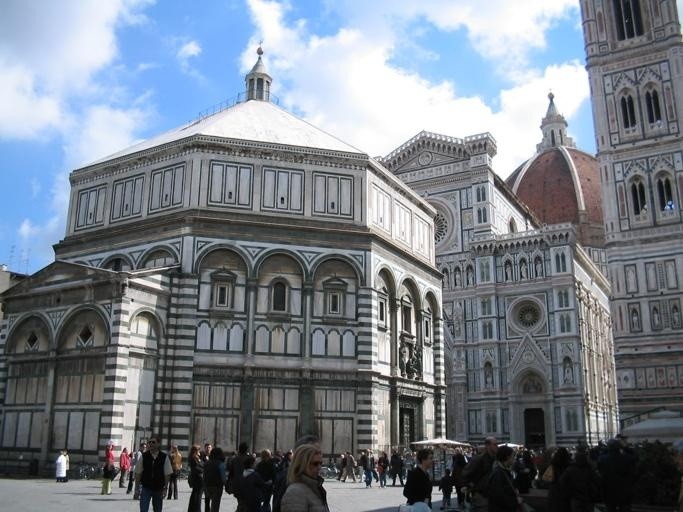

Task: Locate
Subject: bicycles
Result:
[318,461,344,480]
[73,462,107,480]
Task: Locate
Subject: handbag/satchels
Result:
[399,503,433,512]
[376,466,384,473]
[520,502,534,512]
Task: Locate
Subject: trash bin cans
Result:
[29,460,39,476]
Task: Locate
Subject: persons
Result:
[101,436,330,512]
[56,451,66,482]
[64,449,70,481]
[403,437,682,512]
[338,449,405,487]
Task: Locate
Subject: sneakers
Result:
[336,476,406,489]
